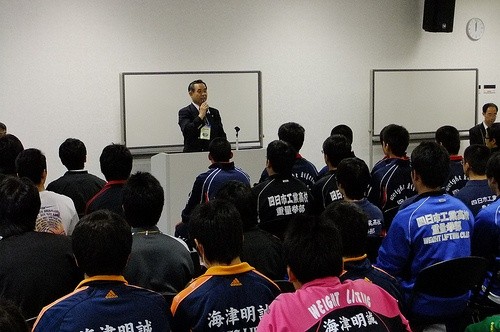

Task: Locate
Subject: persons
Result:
[31,210,170,332]
[88,145,134,213]
[124,172,195,300]
[253,123,419,239]
[175,139,252,243]
[218,180,285,280]
[169,201,281,332]
[437,103,500,307]
[47,138,107,218]
[0,122,81,332]
[179,79,227,153]
[257,218,411,332]
[328,201,402,301]
[370,141,474,321]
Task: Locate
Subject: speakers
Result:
[423,0,455,32]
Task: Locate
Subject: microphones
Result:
[202,99,209,113]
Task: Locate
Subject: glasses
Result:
[460,161,468,165]
[408,162,415,168]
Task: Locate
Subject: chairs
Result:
[407,256,500,332]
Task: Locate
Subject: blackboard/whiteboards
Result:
[368,68,479,141]
[120,71,265,153]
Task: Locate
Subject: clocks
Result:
[466,18,485,41]
